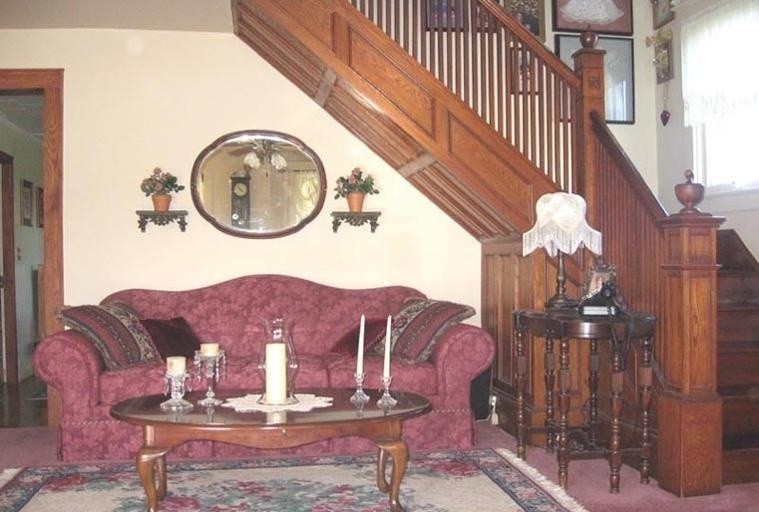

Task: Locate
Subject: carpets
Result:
[1,448,591,510]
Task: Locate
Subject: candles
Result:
[199,339,220,356]
[166,354,187,374]
[381,313,394,380]
[354,310,367,375]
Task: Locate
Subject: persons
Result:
[507,1,538,35]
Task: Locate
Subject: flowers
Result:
[139,166,186,198]
[332,166,382,199]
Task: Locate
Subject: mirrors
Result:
[191,130,326,240]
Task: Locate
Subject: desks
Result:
[513,306,656,490]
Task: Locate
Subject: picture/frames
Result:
[19,176,35,227]
[501,0,547,44]
[36,186,44,228]
[581,261,617,299]
[652,39,674,85]
[651,1,676,30]
[553,32,636,125]
[422,0,500,34]
[550,0,634,36]
[509,46,544,95]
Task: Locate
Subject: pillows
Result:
[133,309,202,366]
[328,318,394,361]
[53,298,166,371]
[363,292,476,367]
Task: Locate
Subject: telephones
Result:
[580,280,618,305]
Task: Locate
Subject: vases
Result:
[149,192,173,212]
[348,192,365,213]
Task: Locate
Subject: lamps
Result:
[239,152,288,171]
[518,187,605,312]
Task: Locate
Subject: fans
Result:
[227,139,297,156]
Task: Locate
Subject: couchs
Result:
[32,271,495,466]
